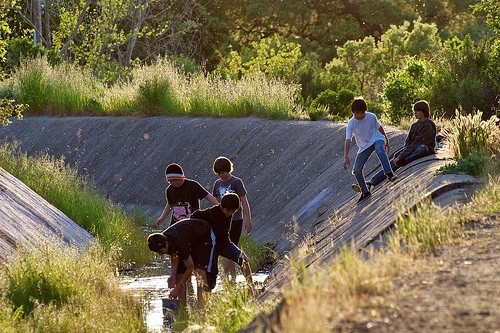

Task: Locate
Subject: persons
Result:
[343,99,398,204]
[148,157,264,308]
[351,100,436,193]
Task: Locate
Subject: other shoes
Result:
[386,171,398,181]
[357,191,372,205]
[352,180,372,192]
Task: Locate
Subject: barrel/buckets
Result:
[162,299,180,316]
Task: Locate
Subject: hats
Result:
[165,163,185,180]
